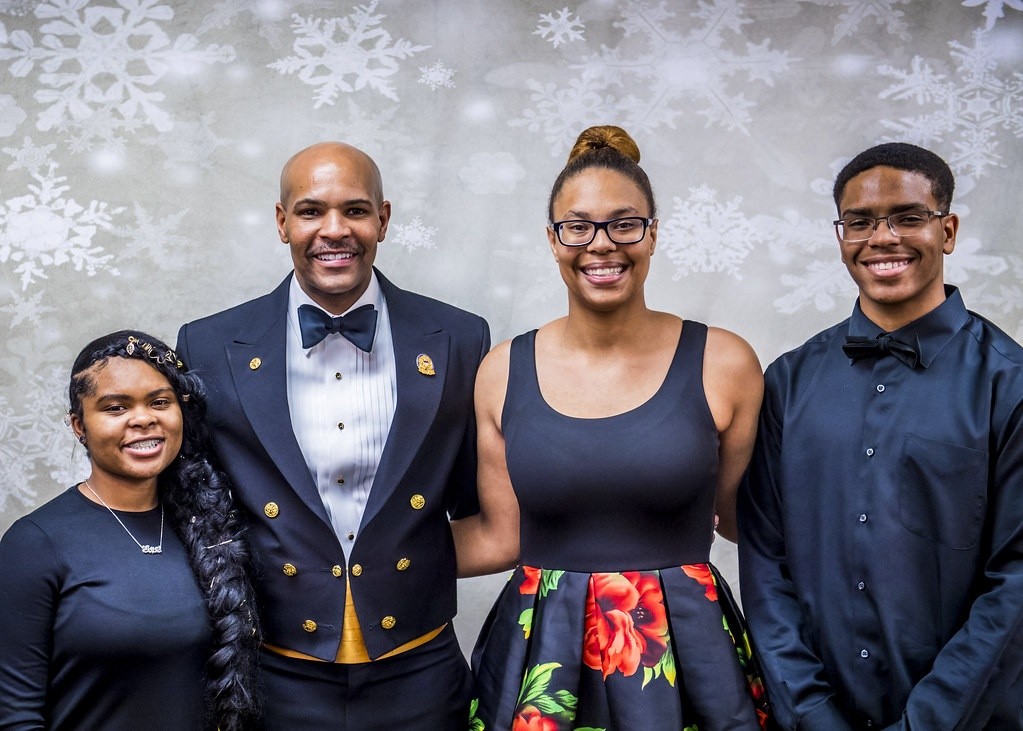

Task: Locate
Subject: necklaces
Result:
[86,480,163,554]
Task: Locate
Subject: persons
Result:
[0,331,260,731]
[446,127,764,731]
[740,143,1022,731]
[174,141,719,731]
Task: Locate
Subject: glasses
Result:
[549,216,654,247]
[833,211,949,242]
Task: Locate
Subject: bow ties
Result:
[842,335,918,369]
[297,303,378,353]
